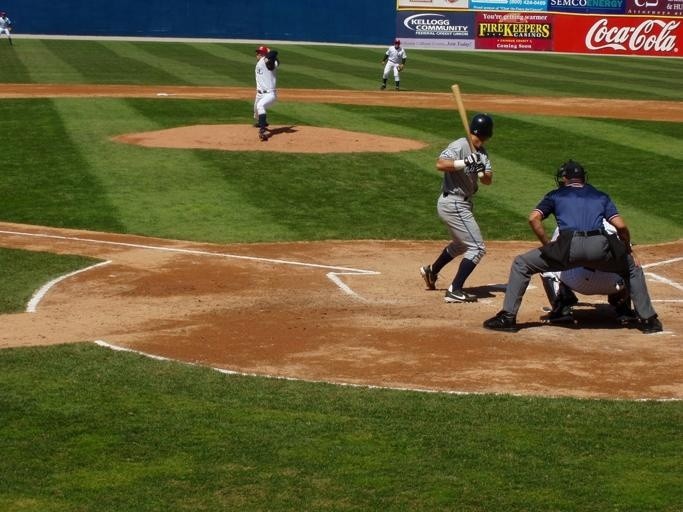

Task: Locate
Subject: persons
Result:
[0,12,14,46]
[539,218,638,327]
[254,46,278,141]
[420,113,494,301]
[484,160,661,334]
[380,41,407,91]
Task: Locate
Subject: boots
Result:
[615,296,639,325]
[540,274,574,323]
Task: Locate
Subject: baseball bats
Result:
[451,84,484,178]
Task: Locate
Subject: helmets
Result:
[469,114,492,141]
[393,39,401,45]
[554,159,587,188]
[255,46,268,55]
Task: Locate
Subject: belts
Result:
[258,90,267,93]
[573,230,606,236]
[443,192,468,201]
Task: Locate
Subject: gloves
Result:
[464,153,480,166]
[476,159,484,173]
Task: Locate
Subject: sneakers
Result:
[259,129,267,140]
[444,288,477,303]
[419,264,437,290]
[483,310,517,332]
[641,313,662,331]
[254,121,269,127]
